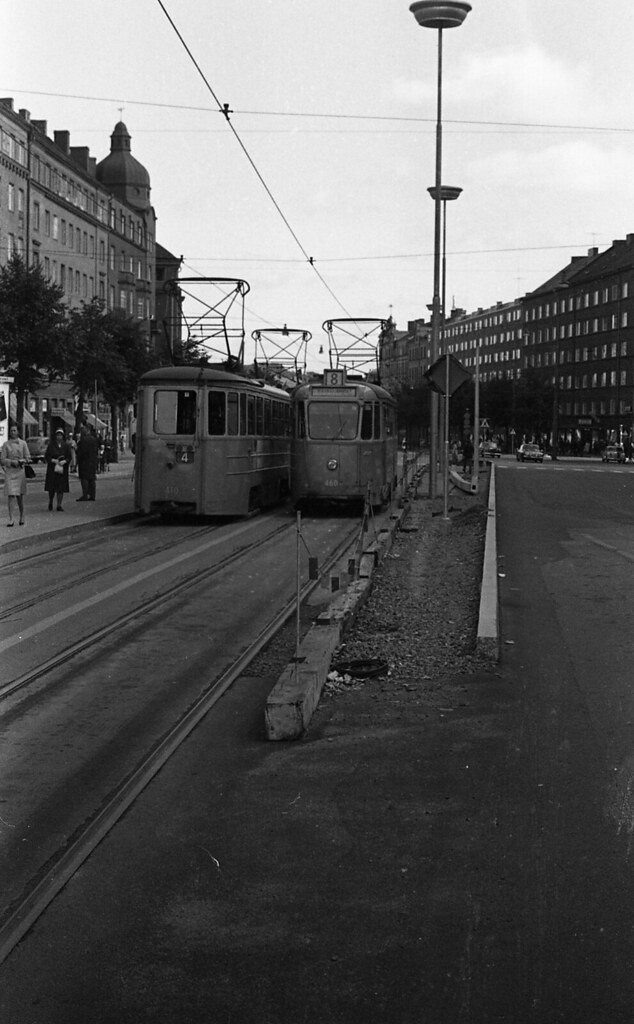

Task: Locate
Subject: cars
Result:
[479,442,501,458]
[516,444,543,463]
[602,446,625,463]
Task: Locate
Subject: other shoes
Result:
[57,506,64,511]
[88,495,95,501]
[76,495,88,501]
[7,521,14,526]
[19,521,24,525]
[49,503,53,510]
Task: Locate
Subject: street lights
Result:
[409,0,472,499]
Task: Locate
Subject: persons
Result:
[41,429,72,513]
[0,423,34,526]
[66,423,128,502]
[449,436,474,474]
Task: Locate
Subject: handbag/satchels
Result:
[54,455,64,474]
[24,461,36,478]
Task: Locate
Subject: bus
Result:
[131,366,399,516]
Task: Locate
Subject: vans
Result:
[26,437,50,455]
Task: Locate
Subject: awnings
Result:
[8,394,37,440]
[59,410,83,428]
[85,413,108,430]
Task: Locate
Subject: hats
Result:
[55,428,64,434]
